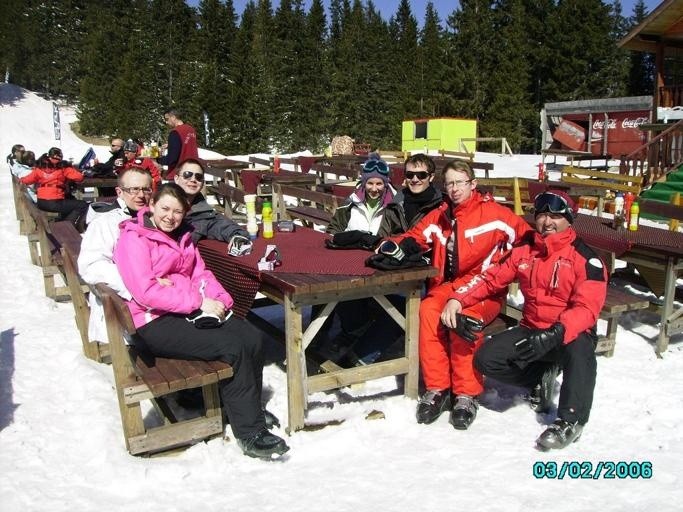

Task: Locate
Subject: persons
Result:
[309,151,405,356]
[173,158,254,257]
[336,152,444,366]
[76,166,153,345]
[154,106,198,183]
[373,157,535,431]
[439,188,610,450]
[5,137,162,229]
[113,183,291,459]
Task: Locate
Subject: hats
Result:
[122,141,137,152]
[543,189,578,218]
[360,152,388,189]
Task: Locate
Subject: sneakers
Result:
[262,408,278,429]
[529,363,559,413]
[236,429,289,460]
[451,393,478,429]
[325,315,373,355]
[175,389,203,409]
[415,388,451,424]
[536,417,583,451]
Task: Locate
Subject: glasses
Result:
[404,170,431,179]
[533,192,567,214]
[182,171,203,182]
[363,160,389,175]
[374,238,399,256]
[265,248,282,267]
[119,186,153,195]
[443,178,475,190]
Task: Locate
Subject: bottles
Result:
[243,193,259,240]
[262,202,274,238]
[612,192,640,232]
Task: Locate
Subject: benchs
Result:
[36,150,683,368]
[47,221,235,450]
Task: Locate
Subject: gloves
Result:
[332,230,379,247]
[514,322,565,363]
[326,237,381,251]
[230,233,251,246]
[371,260,427,270]
[439,312,482,342]
[364,237,419,264]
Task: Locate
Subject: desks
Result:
[200,223,439,436]
